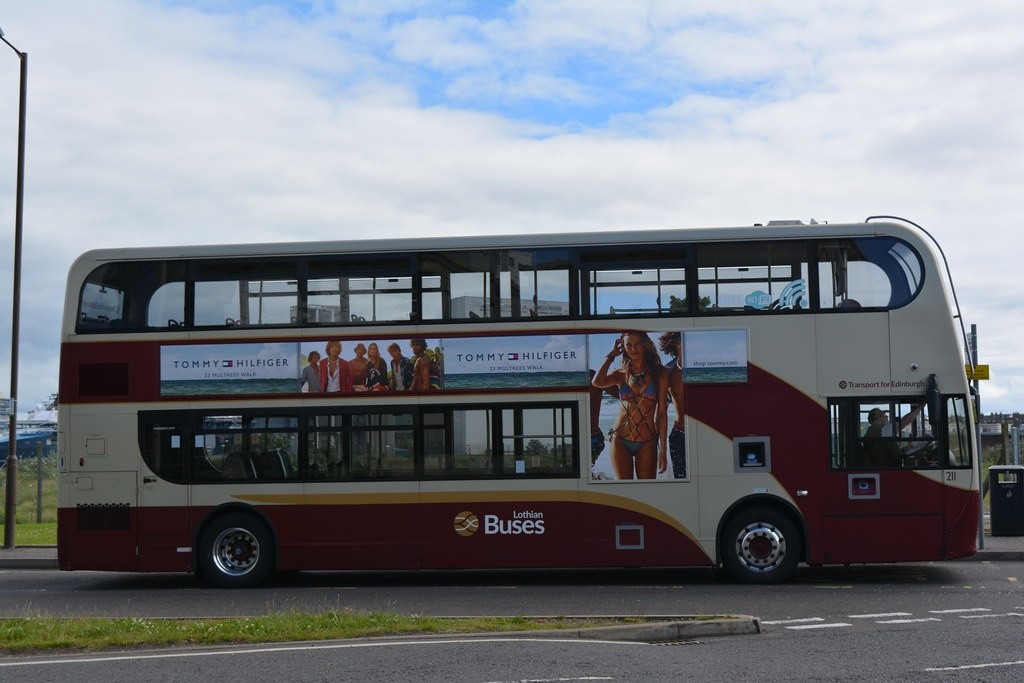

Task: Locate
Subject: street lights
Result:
[0,27,27,548]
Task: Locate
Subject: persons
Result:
[591,332,668,479]
[363,343,388,390]
[319,341,348,393]
[388,344,414,391]
[348,343,369,392]
[301,351,320,392]
[863,399,927,467]
[589,369,619,467]
[408,340,433,392]
[658,332,686,479]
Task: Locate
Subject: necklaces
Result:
[676,358,683,370]
[632,367,646,377]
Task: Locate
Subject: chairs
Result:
[82,305,614,329]
[221,445,296,484]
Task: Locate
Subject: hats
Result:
[354,344,367,355]
[410,339,427,350]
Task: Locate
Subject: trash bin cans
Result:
[988,465,1024,536]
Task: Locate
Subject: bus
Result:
[56,217,981,585]
